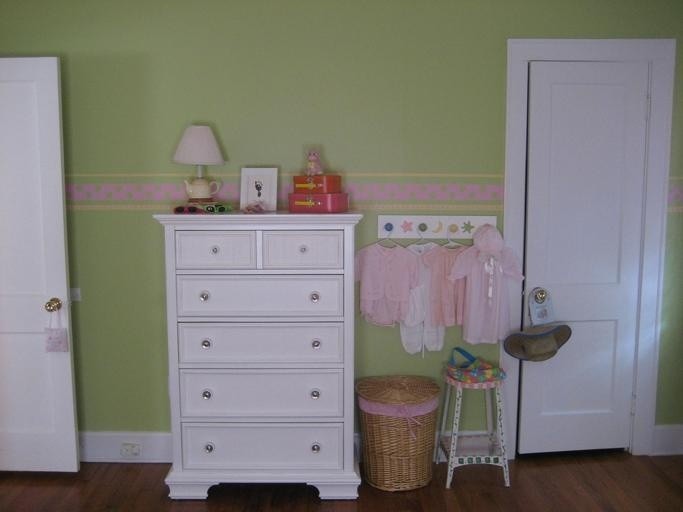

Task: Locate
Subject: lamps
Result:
[170,123,227,206]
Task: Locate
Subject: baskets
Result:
[354,366,442,493]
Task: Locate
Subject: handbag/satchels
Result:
[443,346,508,385]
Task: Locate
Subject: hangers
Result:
[375,220,465,249]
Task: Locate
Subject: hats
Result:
[500,320,574,364]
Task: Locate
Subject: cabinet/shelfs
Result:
[149,211,364,499]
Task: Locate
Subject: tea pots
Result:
[182,177,221,198]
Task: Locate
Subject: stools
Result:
[436,366,514,489]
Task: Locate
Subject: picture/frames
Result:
[239,168,277,213]
